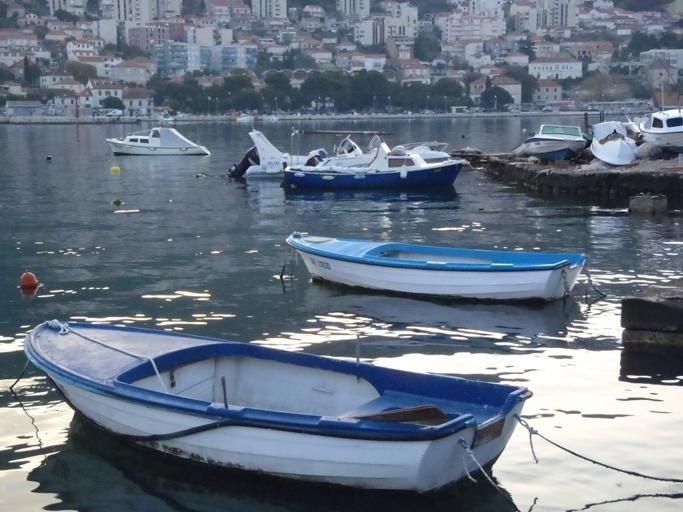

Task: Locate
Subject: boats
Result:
[23,319,534,496]
[105,126,212,155]
[283,231,591,303]
